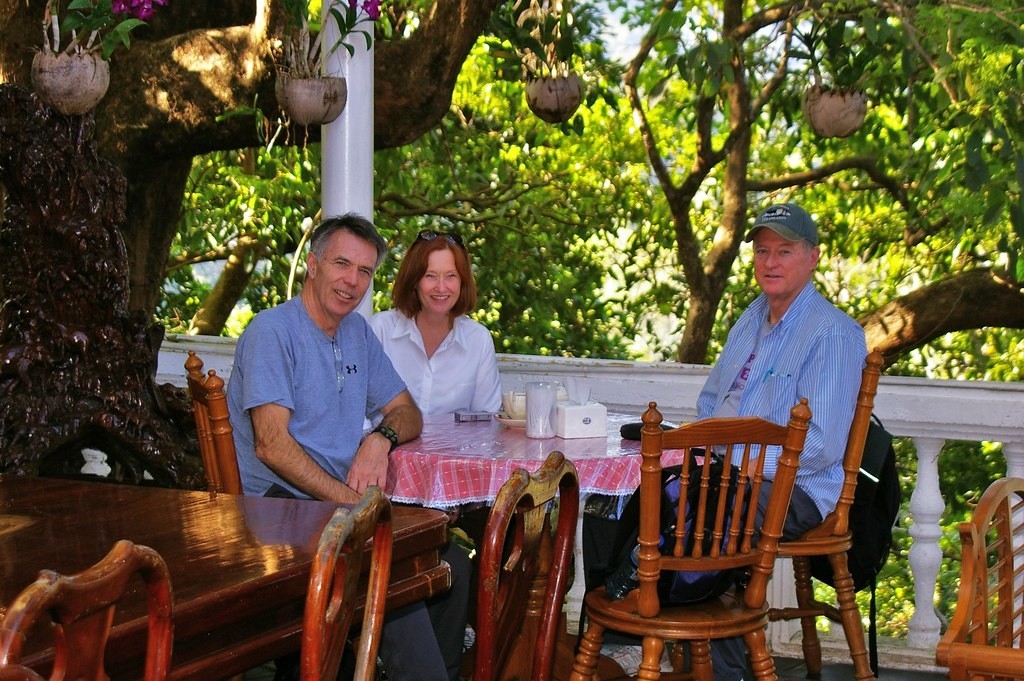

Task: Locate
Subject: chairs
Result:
[569,397,813,681]
[469,450,579,681]
[935,477,1024,681]
[0,538,175,680]
[185,348,362,681]
[300,485,393,681]
[766,348,885,681]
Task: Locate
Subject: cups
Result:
[503,391,525,419]
[556,386,568,401]
[525,381,558,439]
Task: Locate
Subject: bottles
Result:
[605,535,664,601]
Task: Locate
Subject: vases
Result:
[523,74,585,122]
[31,50,111,116]
[274,73,348,126]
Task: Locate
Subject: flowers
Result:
[484,0,591,79]
[269,0,385,76]
[32,0,169,61]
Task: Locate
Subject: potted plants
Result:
[780,12,900,138]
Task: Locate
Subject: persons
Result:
[581,203,866,681]
[366,229,503,578]
[228,215,470,681]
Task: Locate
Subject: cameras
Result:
[454,410,493,422]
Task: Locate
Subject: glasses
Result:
[415,230,464,250]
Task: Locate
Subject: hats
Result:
[745,203,818,246]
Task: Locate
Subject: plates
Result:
[494,413,526,430]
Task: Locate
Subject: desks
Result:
[0,471,453,681]
[362,415,715,681]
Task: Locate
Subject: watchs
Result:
[371,427,397,451]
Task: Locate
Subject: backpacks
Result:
[812,413,900,594]
[605,447,753,605]
